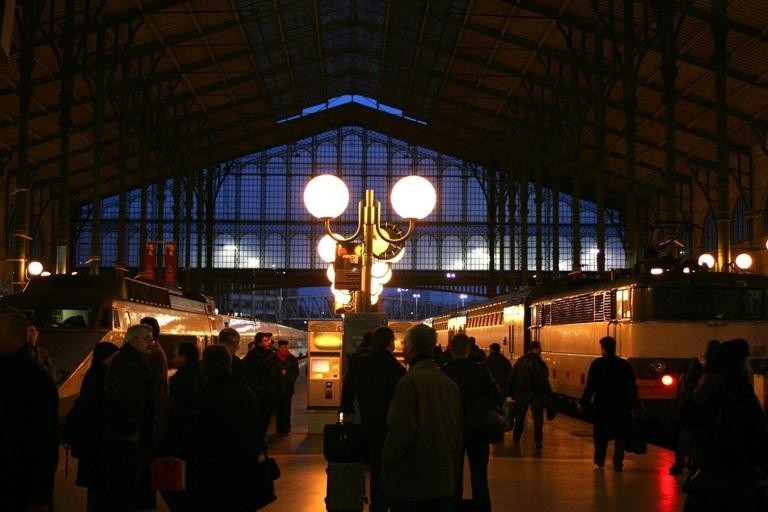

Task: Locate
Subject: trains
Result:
[425,267,764,426]
[25,267,308,411]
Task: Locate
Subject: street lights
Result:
[294,167,435,311]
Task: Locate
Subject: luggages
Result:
[325,466,368,512]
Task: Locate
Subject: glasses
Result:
[140,336,156,344]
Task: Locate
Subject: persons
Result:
[342,325,513,511]
[669,338,768,511]
[507,342,549,449]
[1,306,298,510]
[577,336,637,472]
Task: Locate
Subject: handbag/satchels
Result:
[151,456,185,490]
[625,417,646,455]
[502,401,516,432]
[323,422,362,463]
[546,393,565,421]
[252,451,280,509]
